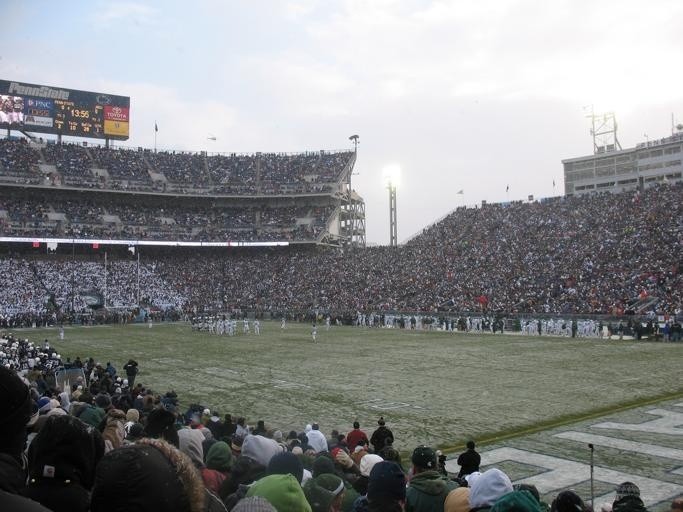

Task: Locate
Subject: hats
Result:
[144,406,176,434]
[617,482,640,498]
[72,376,144,438]
[272,430,306,454]
[670,499,683,511]
[312,446,482,512]
[0,364,32,428]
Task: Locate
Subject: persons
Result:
[0,136,683,342]
[0,94,24,123]
[671,497,683,512]
[612,481,646,512]
[0,333,585,512]
